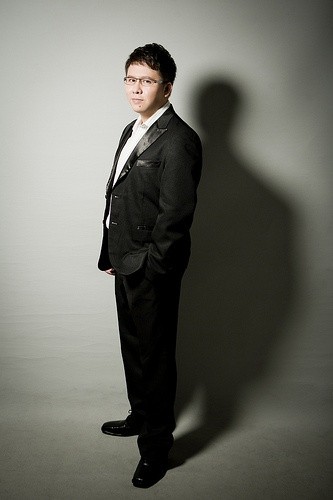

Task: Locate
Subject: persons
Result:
[97,43,203,489]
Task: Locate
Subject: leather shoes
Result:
[100,414,140,438]
[131,456,167,488]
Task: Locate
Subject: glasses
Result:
[123,76,163,87]
